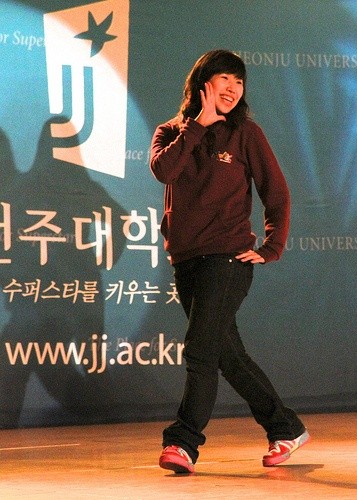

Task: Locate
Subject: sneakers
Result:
[263,427,310,466]
[158,445,194,473]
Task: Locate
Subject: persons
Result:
[148,48,313,474]
